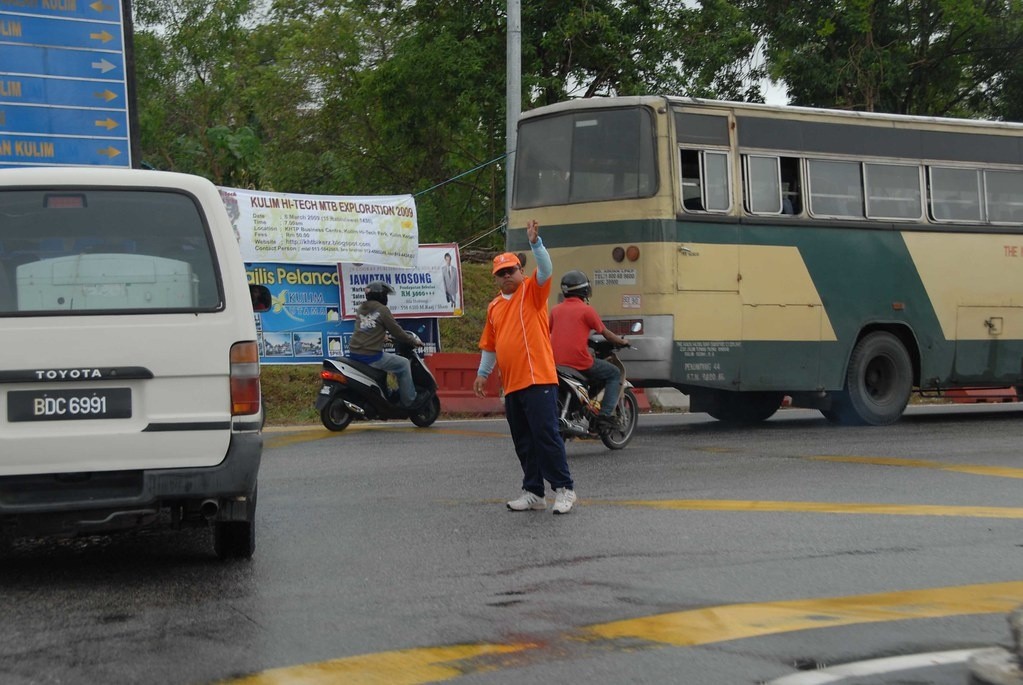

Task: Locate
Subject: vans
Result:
[0,166,274,563]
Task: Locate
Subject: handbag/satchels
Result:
[446,293,450,302]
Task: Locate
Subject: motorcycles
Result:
[553,321,643,450]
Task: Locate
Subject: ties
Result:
[448,266,451,278]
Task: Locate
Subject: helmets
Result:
[560,270,593,298]
[365,281,393,294]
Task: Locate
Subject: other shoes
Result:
[598,414,617,424]
[403,390,430,409]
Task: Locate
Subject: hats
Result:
[491,252,521,274]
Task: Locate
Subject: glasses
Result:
[495,267,520,277]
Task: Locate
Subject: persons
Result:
[347,279,430,407]
[473,218,577,514]
[441,252,458,308]
[548,268,630,428]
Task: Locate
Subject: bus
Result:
[504,93,1023,427]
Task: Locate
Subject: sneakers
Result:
[506,488,547,510]
[553,486,576,514]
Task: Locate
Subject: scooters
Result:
[314,321,443,432]
